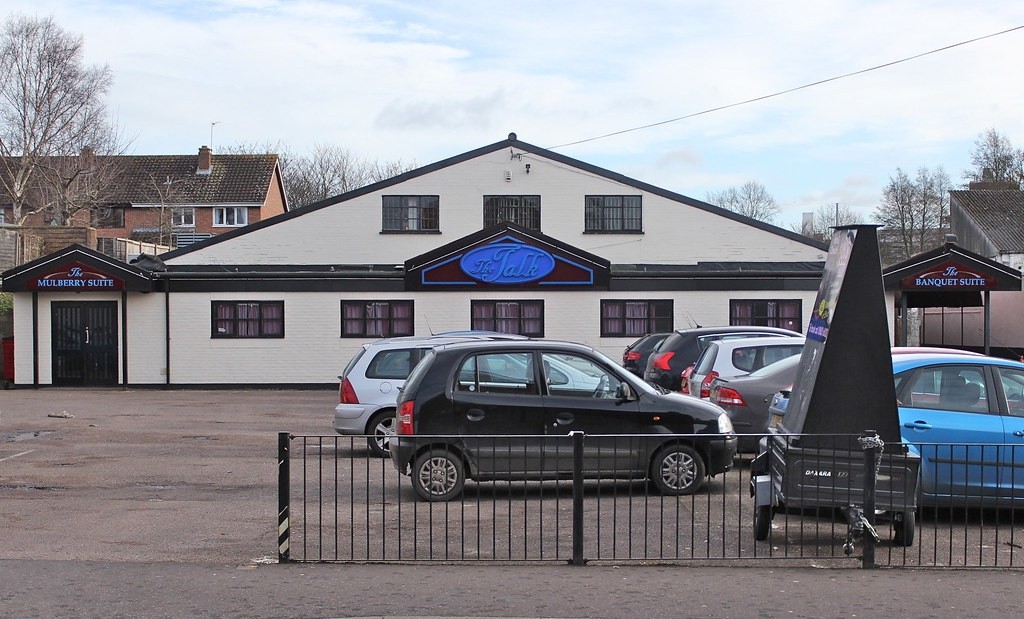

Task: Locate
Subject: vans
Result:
[333,338,603,459]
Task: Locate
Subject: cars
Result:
[708,346,1024,455]
[681,361,697,396]
[766,353,1024,510]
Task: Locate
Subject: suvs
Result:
[643,311,806,394]
[429,330,614,397]
[387,340,739,502]
[688,337,806,402]
[622,332,675,382]
